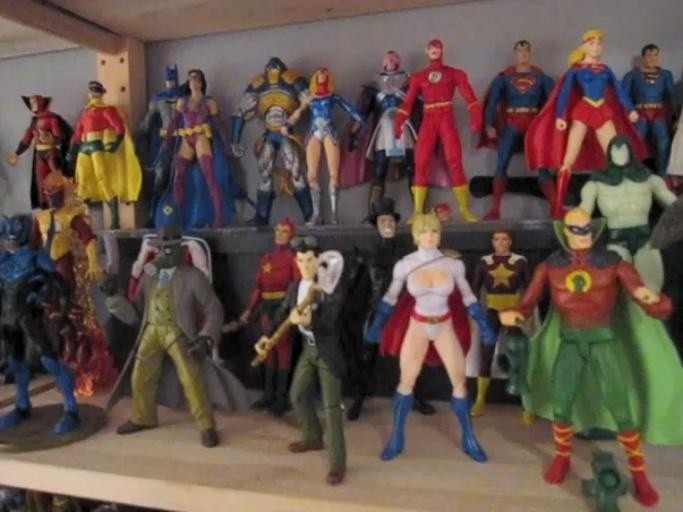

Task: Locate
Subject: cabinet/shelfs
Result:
[0,0,682,512]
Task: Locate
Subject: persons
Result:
[0,29,683,512]
